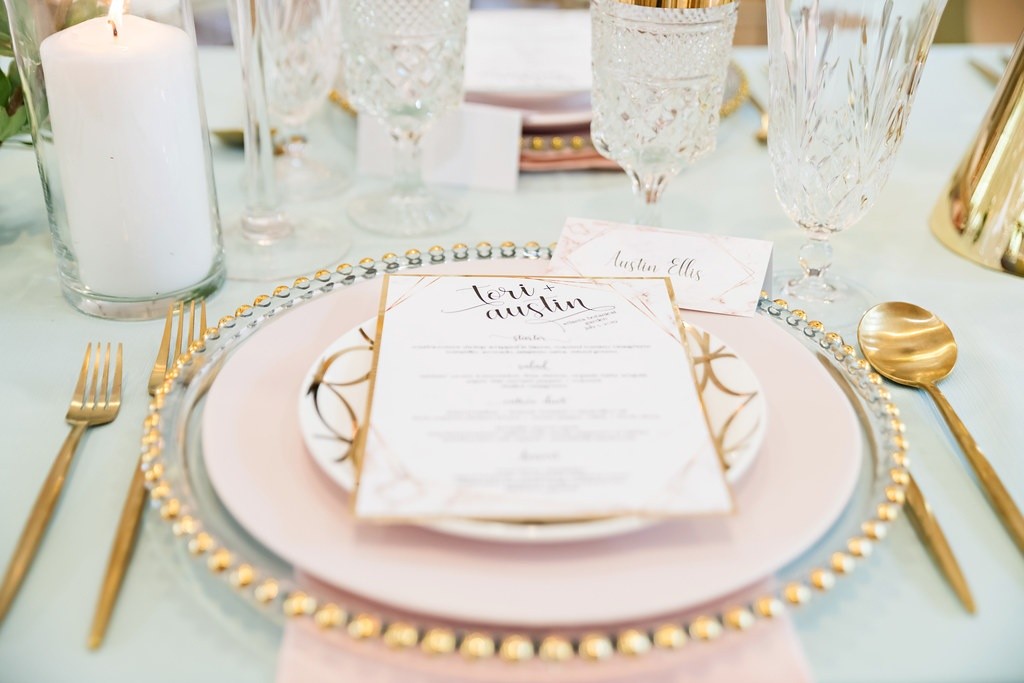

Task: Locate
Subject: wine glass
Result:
[589,0,739,232]
[228,0,477,280]
[761,0,948,330]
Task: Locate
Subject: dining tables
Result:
[0,41,1024,681]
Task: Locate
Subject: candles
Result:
[39,0,222,299]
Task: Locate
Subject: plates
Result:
[296,316,767,547]
[463,7,591,104]
[511,100,587,132]
[201,261,863,628]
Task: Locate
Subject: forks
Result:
[0,342,123,622]
[89,300,206,647]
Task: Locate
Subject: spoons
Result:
[857,301,1024,555]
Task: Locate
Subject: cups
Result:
[5,0,229,321]
[929,23,1024,281]
